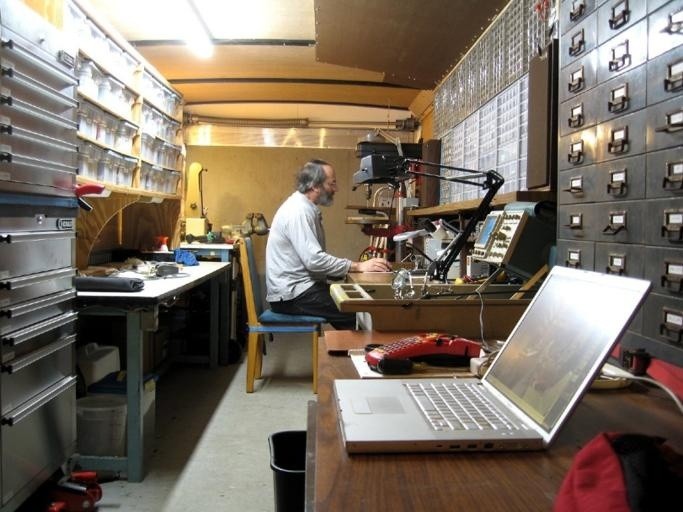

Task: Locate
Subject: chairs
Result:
[240,236,329,394]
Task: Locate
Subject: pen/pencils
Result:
[328,348,369,356]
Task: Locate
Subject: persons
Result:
[265,159,393,330]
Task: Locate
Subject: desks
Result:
[346,260,444,284]
[71,242,237,484]
[303,336,683,512]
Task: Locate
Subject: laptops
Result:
[332,265,653,454]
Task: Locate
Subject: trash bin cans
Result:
[76,395,127,483]
[269,430,307,512]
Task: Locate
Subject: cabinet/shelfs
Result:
[62,0,185,202]
[555,1,683,372]
[404,190,552,280]
